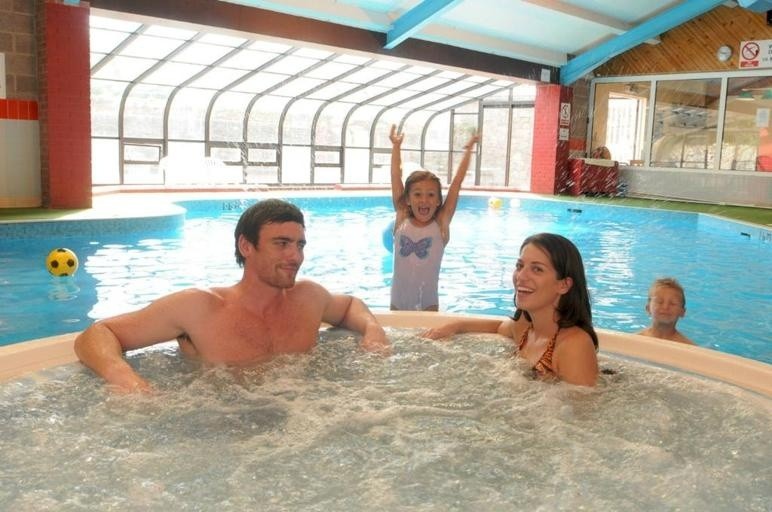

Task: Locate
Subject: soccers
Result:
[489,197,502,208]
[46,248,78,275]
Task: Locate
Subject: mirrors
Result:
[583,69,771,177]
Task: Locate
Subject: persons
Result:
[634,277,696,349]
[414,232,600,386]
[389,124,483,313]
[73,199,399,400]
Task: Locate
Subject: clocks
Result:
[715,43,733,62]
